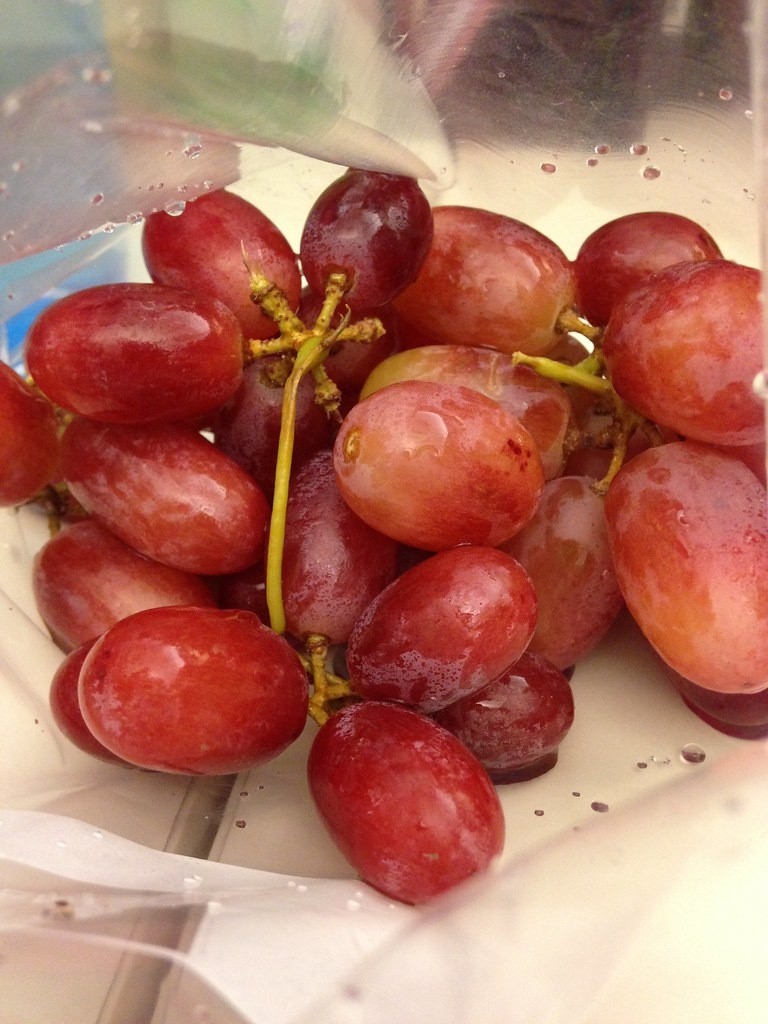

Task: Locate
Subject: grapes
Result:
[0,165,768,908]
